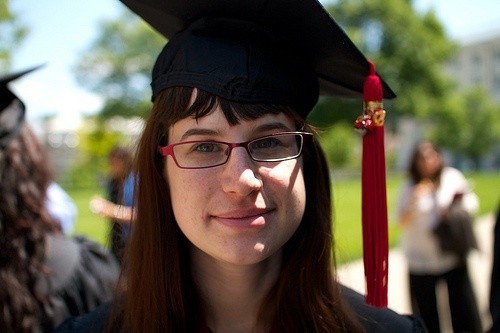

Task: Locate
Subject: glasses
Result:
[158,131,315,169]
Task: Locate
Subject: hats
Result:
[119,0,397,309]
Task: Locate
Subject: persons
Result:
[91,144,141,266]
[397,139,483,333]
[0,74,124,333]
[55,14,420,333]
